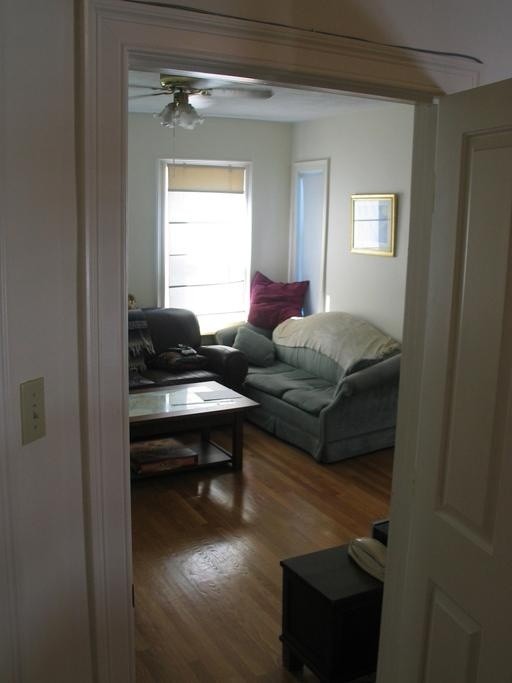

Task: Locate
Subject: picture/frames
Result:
[350,194,397,257]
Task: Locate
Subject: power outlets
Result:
[20,376,47,445]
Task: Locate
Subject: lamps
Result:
[155,74,206,131]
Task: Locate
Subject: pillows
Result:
[232,272,400,379]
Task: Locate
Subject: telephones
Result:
[347,537,387,582]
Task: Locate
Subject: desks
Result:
[281,543,381,682]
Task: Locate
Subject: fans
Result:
[126,72,275,104]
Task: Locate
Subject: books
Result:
[130,437,200,474]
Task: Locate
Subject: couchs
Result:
[129,307,248,393]
[199,321,401,466]
